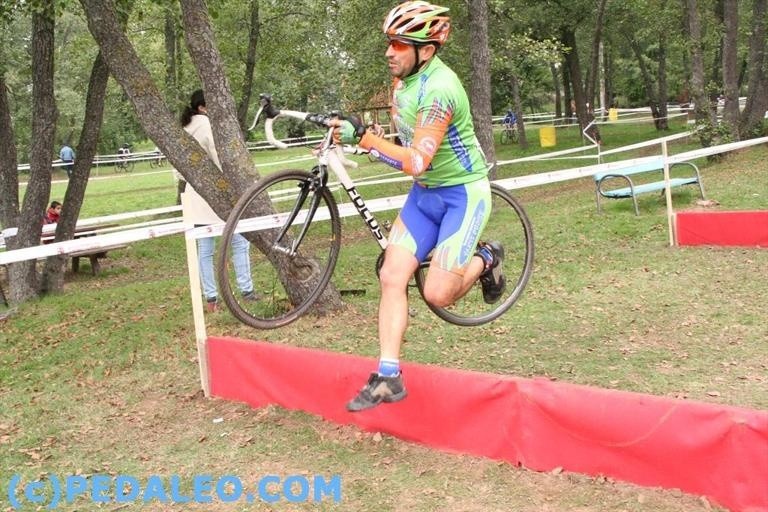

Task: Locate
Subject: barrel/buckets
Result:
[539,127,556,147]
[608,108,617,121]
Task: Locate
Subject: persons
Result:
[117,143,134,173]
[41,201,62,245]
[326,1,507,413]
[169,88,269,312]
[59,143,75,179]
[153,148,167,161]
[361,120,387,137]
[501,108,517,137]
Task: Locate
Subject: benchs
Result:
[596,158,705,214]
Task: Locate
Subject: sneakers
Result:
[241,290,261,303]
[480,240,506,304]
[207,297,224,312]
[346,369,408,411]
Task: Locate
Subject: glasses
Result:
[383,36,438,51]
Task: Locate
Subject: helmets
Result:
[383,0,451,45]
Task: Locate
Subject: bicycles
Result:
[217,93,534,328]
[114,143,135,173]
[498,111,520,145]
[150,151,168,170]
